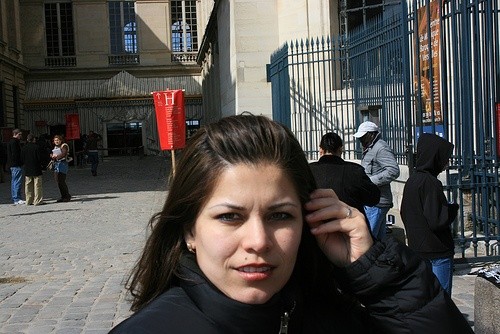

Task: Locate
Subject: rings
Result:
[347,208,352,218]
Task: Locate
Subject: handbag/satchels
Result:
[53,157,68,175]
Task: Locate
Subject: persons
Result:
[308,133,380,245]
[400,133,460,300]
[105,111,474,334]
[7,128,104,206]
[352,121,400,243]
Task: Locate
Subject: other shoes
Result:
[57,195,71,202]
[30,201,46,205]
[92,171,97,176]
[13,199,26,205]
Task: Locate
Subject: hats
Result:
[352,121,379,138]
